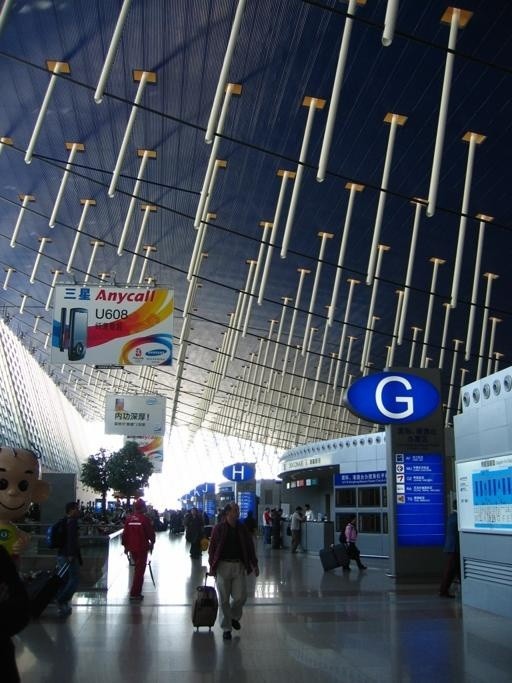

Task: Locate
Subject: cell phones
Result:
[59,308,66,352]
[68,308,88,360]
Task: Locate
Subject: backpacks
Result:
[339,530,346,543]
[46,518,67,549]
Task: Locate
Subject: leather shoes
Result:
[223,631,230,639]
[232,620,240,629]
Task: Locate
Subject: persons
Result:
[0,544,73,682]
[439,499,460,598]
[244,510,258,553]
[208,503,260,640]
[147,504,223,559]
[49,502,84,618]
[122,498,155,600]
[0,445,51,576]
[342,515,369,571]
[262,502,316,553]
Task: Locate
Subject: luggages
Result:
[192,573,219,631]
[29,560,74,618]
[320,544,350,571]
